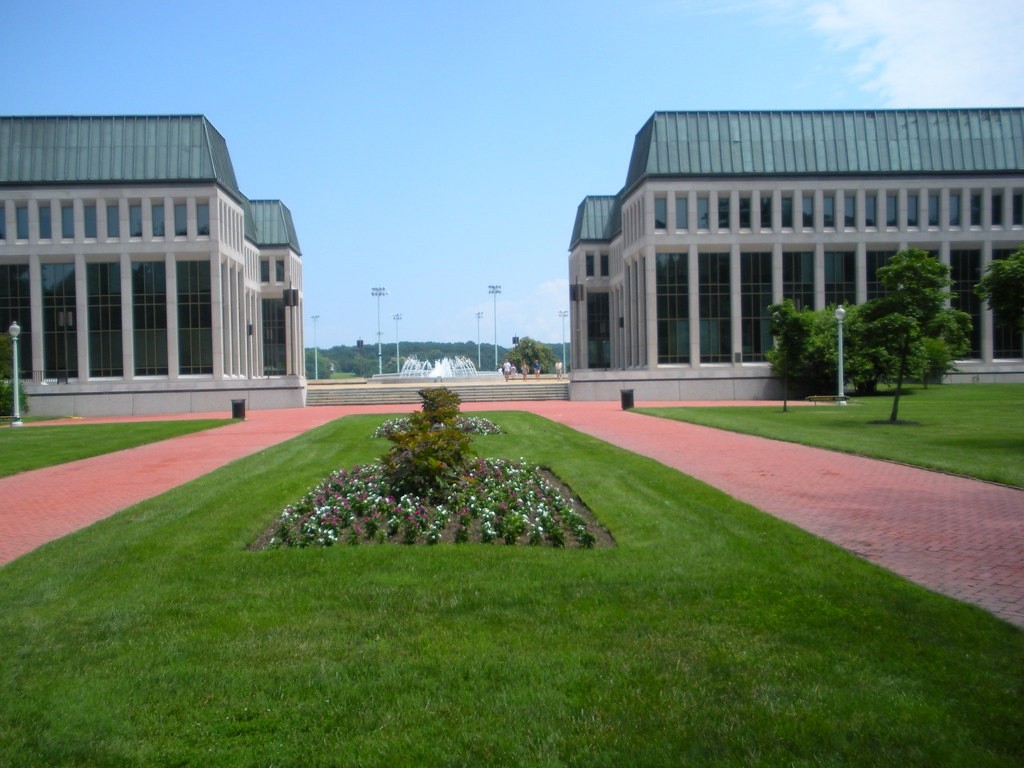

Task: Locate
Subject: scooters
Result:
[511,367,518,381]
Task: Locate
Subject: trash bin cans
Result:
[230,398,246,419]
[621,388,635,411]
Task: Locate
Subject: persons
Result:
[555,359,562,381]
[502,359,512,382]
[522,363,529,382]
[533,359,541,379]
[510,363,517,379]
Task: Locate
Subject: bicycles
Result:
[534,368,541,381]
[504,366,510,382]
[522,368,529,382]
[556,369,563,381]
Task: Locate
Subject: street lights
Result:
[488,285,502,371]
[310,313,320,379]
[835,305,847,405]
[559,311,568,374]
[8,320,23,427]
[475,312,484,369]
[392,313,403,374]
[371,286,390,373]
